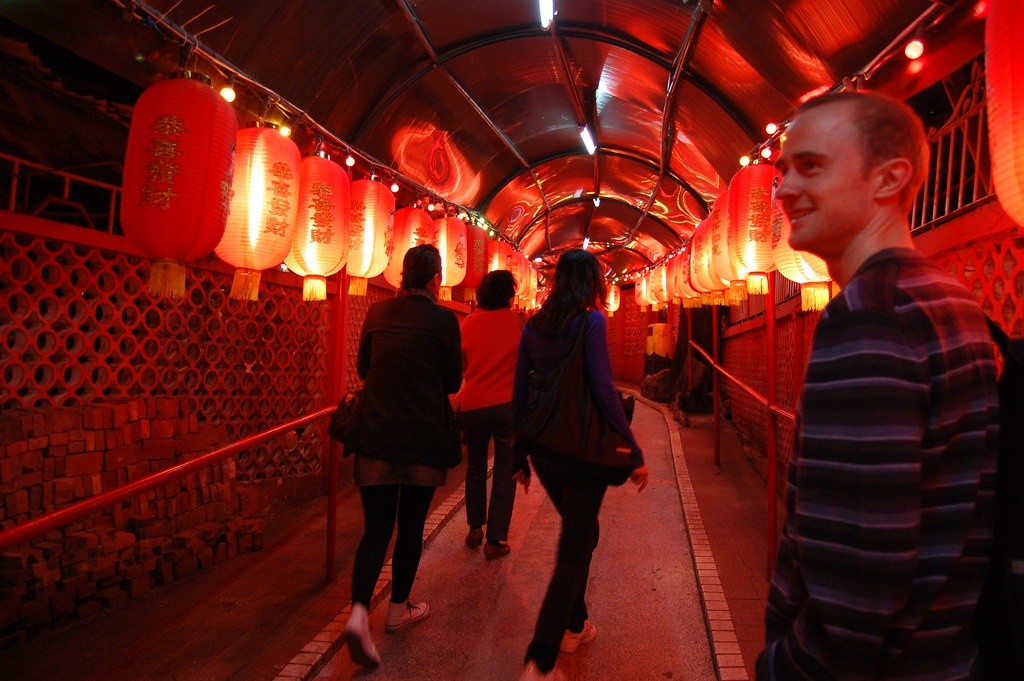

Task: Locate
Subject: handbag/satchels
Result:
[974,310,1024,680]
[518,309,628,486]
[328,392,362,440]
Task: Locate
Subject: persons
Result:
[342,244,463,666]
[755,92,1024,681]
[510,248,649,681]
[459,270,525,561]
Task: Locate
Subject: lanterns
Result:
[120,0,1024,312]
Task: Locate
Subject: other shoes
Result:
[465,530,484,548]
[483,541,510,560]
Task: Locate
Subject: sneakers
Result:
[345,603,381,668]
[559,618,596,652]
[384,600,431,633]
[519,658,566,681]
